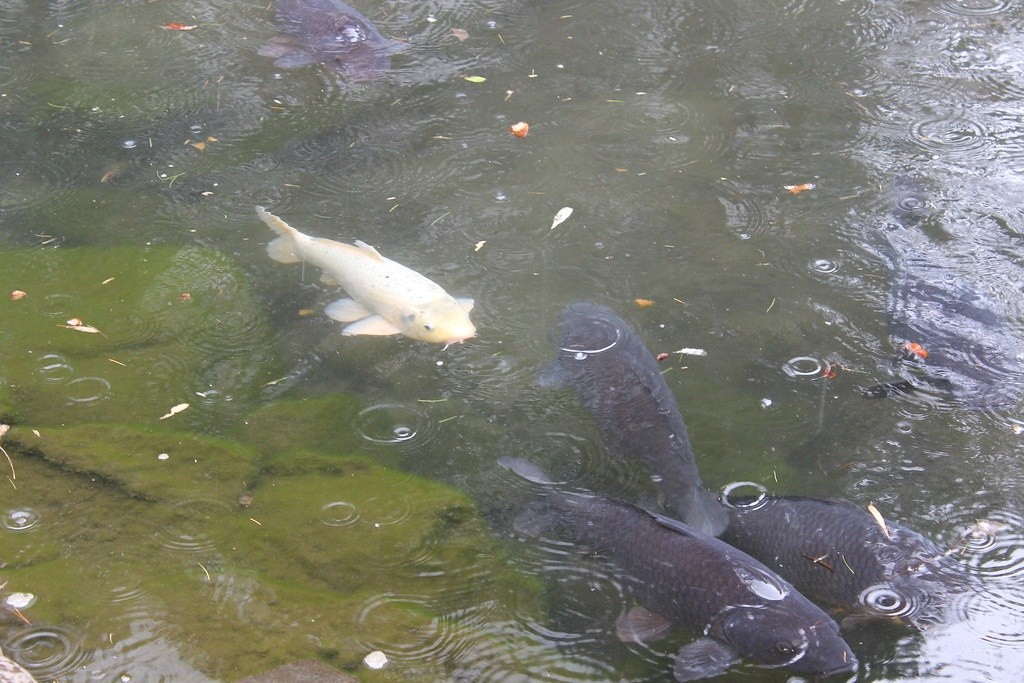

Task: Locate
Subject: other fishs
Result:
[1,1,392,218]
[478,150,1022,683]
[252,204,478,352]
